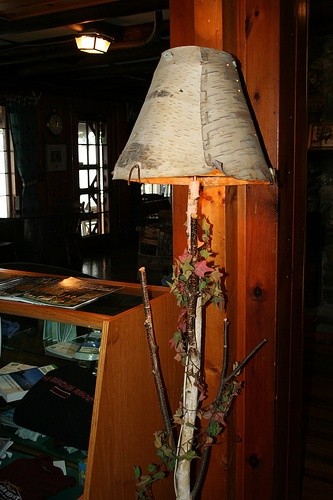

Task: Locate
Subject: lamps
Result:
[75,31,114,54]
[113,45,276,498]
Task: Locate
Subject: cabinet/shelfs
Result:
[0,267,192,500]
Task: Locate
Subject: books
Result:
[0,274,124,310]
[0,362,58,403]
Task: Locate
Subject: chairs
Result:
[135,222,172,286]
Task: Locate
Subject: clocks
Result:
[46,111,65,136]
